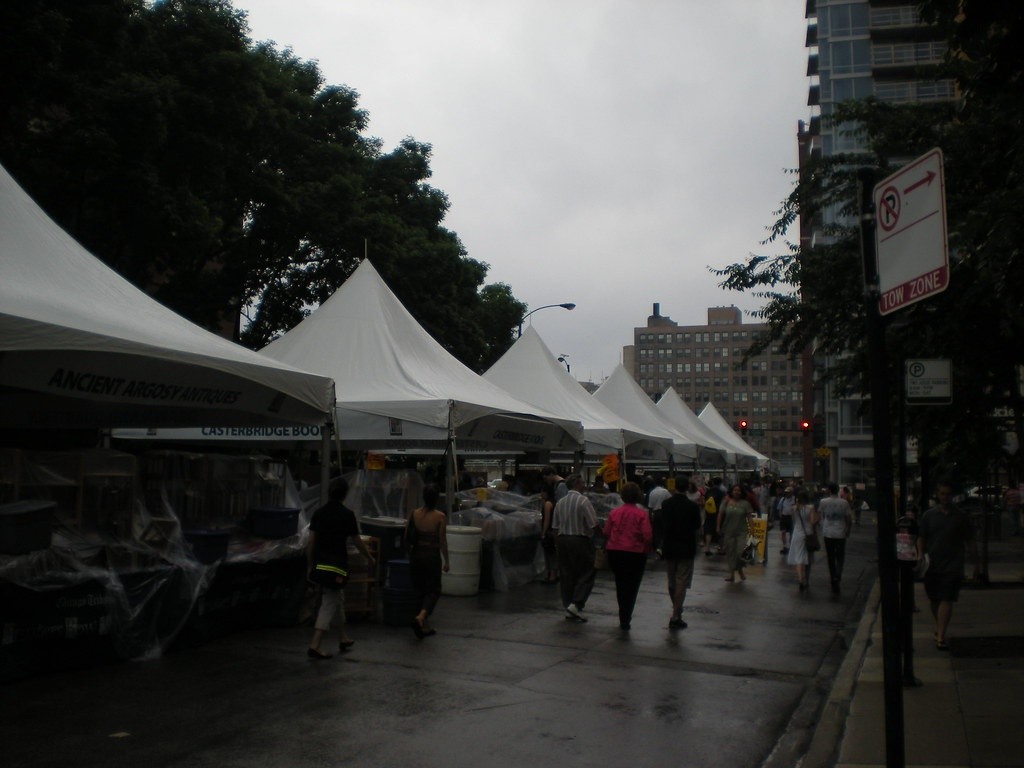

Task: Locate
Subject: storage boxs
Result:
[182,529,233,564]
[242,507,303,538]
[0,499,59,556]
[344,514,418,627]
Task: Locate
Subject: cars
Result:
[954,485,1010,504]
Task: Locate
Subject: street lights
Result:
[558,357,569,373]
[518,304,576,338]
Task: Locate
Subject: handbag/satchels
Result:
[805,534,820,553]
[533,541,550,582]
[405,511,418,545]
[914,553,930,578]
[742,535,759,560]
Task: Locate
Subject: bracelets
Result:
[370,559,374,560]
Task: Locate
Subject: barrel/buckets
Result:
[386,560,421,626]
[440,524,481,597]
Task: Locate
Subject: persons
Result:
[306,479,375,659]
[705,475,861,595]
[1001,478,1021,535]
[539,466,702,630]
[405,485,449,639]
[918,481,980,649]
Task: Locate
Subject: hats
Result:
[784,487,794,492]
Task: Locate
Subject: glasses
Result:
[936,492,953,497]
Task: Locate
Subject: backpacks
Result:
[704,491,719,513]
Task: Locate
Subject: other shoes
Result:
[422,627,436,637]
[307,648,334,659]
[621,624,630,630]
[339,639,355,651]
[1007,530,1020,536]
[566,601,588,622]
[912,607,921,613]
[705,548,845,601]
[669,619,688,630]
[934,629,940,641]
[935,641,949,650]
[410,617,425,639]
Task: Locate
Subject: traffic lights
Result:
[741,420,747,434]
[802,419,810,436]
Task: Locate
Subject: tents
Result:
[0,161,771,526]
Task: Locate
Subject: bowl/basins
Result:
[461,500,478,507]
[480,502,494,508]
